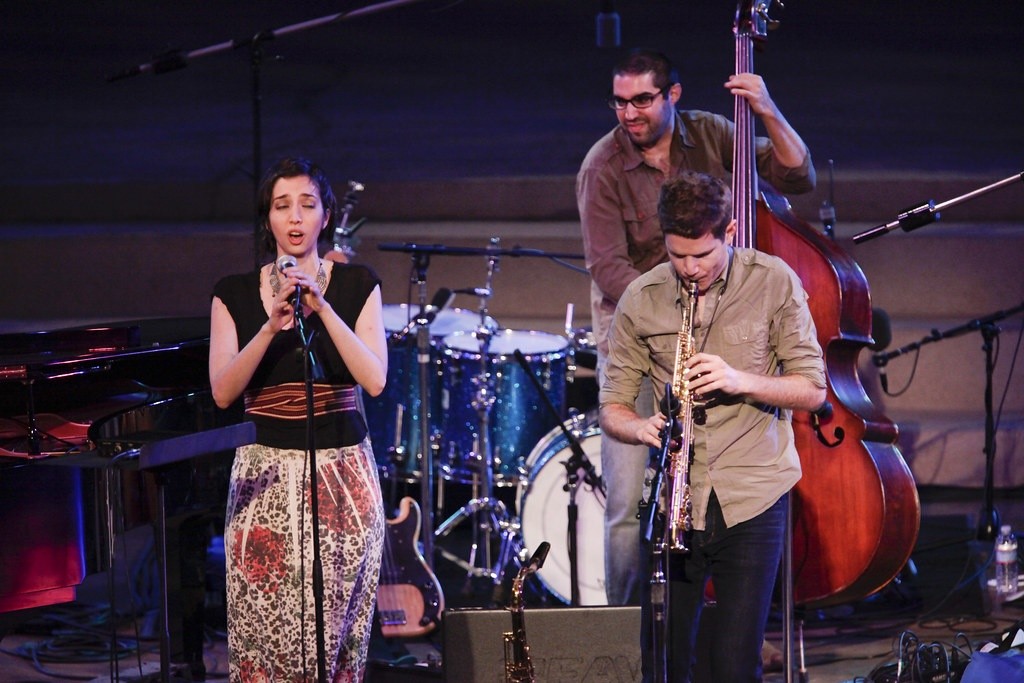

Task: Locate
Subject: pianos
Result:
[1,308,248,629]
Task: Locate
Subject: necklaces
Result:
[269,258,328,297]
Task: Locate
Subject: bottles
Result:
[994,525,1019,594]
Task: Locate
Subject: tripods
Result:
[886,298,1024,569]
[433,325,550,608]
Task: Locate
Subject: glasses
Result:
[606,82,675,110]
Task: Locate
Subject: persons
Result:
[208,156,388,683]
[576,47,817,671]
[598,172,828,683]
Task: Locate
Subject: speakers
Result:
[440,605,655,683]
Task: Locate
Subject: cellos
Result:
[723,0,920,682]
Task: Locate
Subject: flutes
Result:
[653,280,704,559]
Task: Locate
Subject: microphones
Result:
[422,287,457,325]
[660,383,681,417]
[276,255,301,296]
[871,308,893,395]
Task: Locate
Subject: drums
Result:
[514,409,664,606]
[361,301,506,484]
[438,327,572,490]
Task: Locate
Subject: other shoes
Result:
[760,638,784,672]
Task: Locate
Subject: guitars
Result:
[371,495,445,641]
